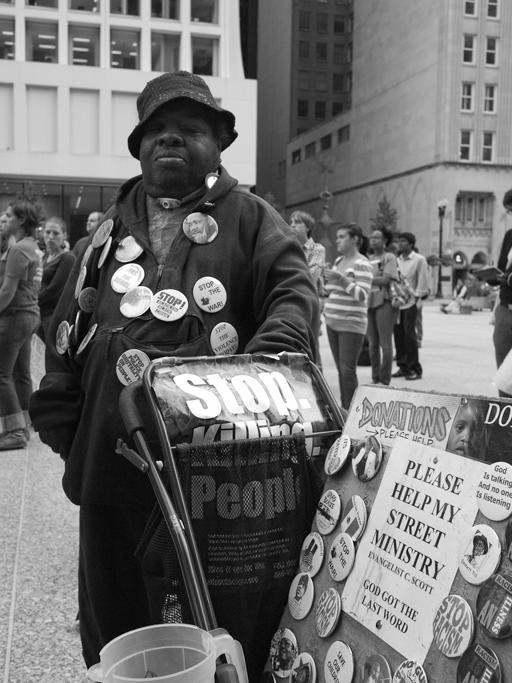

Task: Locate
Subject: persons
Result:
[465,535,489,565]
[273,630,296,670]
[289,190,434,410]
[446,400,493,461]
[483,188,512,398]
[293,575,308,604]
[185,212,216,243]
[292,667,311,683]
[0,199,104,451]
[353,440,376,480]
[440,278,467,314]
[28,70,320,670]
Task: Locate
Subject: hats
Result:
[127,70,238,162]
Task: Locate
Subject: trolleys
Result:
[108,340,356,683]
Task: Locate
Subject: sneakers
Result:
[0,428,30,450]
[391,366,423,379]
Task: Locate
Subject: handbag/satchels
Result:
[380,252,416,309]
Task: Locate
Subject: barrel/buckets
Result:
[79,624,248,683]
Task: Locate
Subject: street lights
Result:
[311,189,342,255]
[434,197,448,300]
